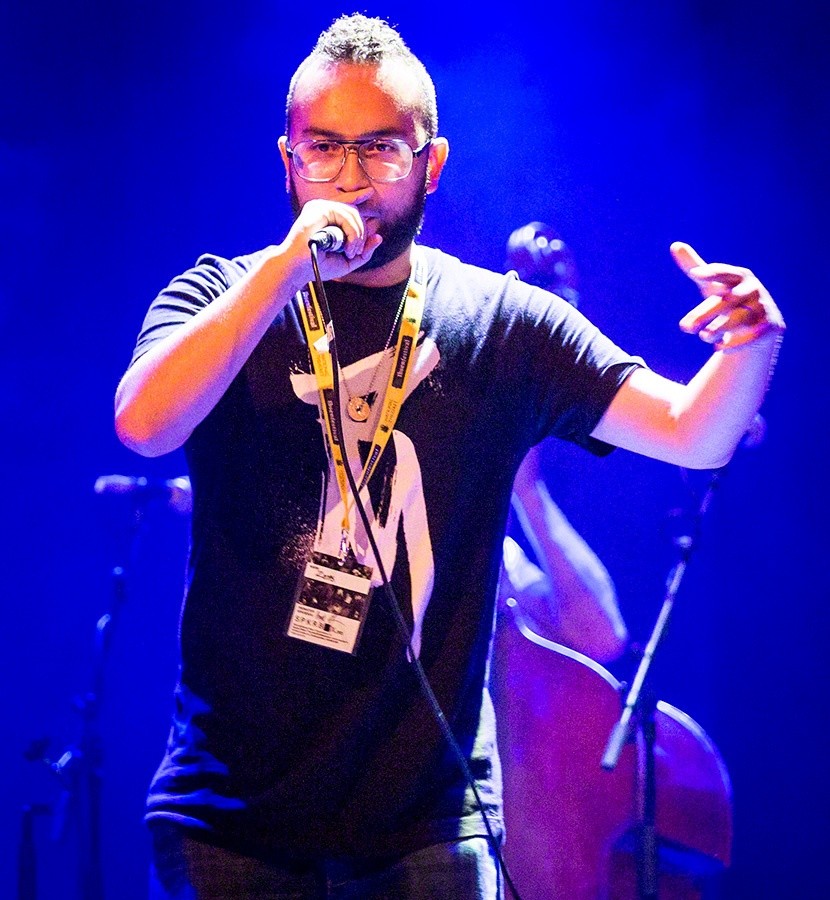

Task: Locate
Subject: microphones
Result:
[307,225,347,252]
[708,410,768,491]
[94,475,191,512]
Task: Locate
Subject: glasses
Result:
[286,138,431,182]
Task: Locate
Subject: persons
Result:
[115,13,787,900]
[501,442,626,662]
